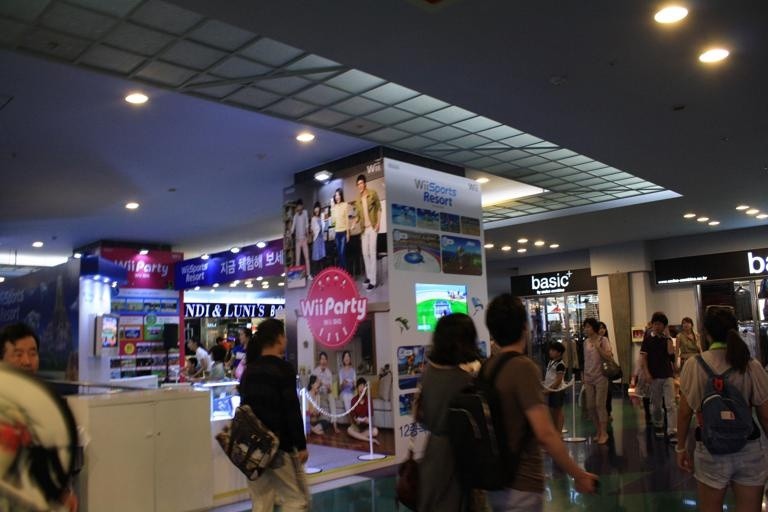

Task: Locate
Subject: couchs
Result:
[298,375,393,430]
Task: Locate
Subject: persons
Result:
[300,351,381,442]
[176,325,250,384]
[352,174,382,291]
[290,199,313,281]
[236,318,311,512]
[542,312,701,447]
[331,188,351,273]
[310,201,326,276]
[480,292,599,512]
[0,319,90,512]
[674,304,768,511]
[411,313,492,512]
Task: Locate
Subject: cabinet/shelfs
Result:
[66,381,241,512]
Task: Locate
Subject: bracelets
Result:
[675,444,689,454]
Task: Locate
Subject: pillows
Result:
[377,374,392,401]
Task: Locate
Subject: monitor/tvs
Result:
[93,312,121,357]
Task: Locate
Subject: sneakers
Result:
[306,274,314,281]
[590,416,613,444]
[667,430,679,444]
[366,284,374,292]
[656,409,664,428]
[362,279,370,285]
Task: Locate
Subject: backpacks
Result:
[445,350,535,491]
[694,352,754,455]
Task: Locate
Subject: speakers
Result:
[163,324,178,347]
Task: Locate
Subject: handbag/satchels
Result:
[397,459,419,512]
[215,405,280,481]
[235,356,247,380]
[599,336,623,381]
